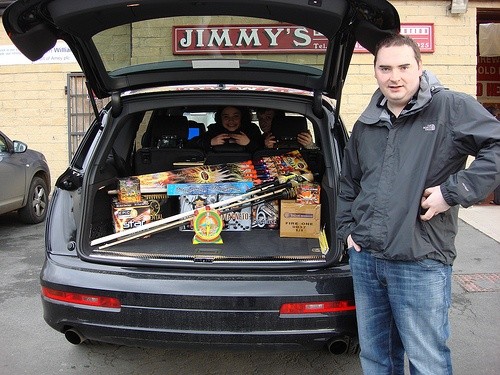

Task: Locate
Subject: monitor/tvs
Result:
[187,124,203,141]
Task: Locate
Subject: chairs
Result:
[136,116,203,174]
[253,116,321,179]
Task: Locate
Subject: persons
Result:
[334,35,500,375]
[256,107,313,148]
[191,106,261,151]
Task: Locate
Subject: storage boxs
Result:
[112,150,321,238]
